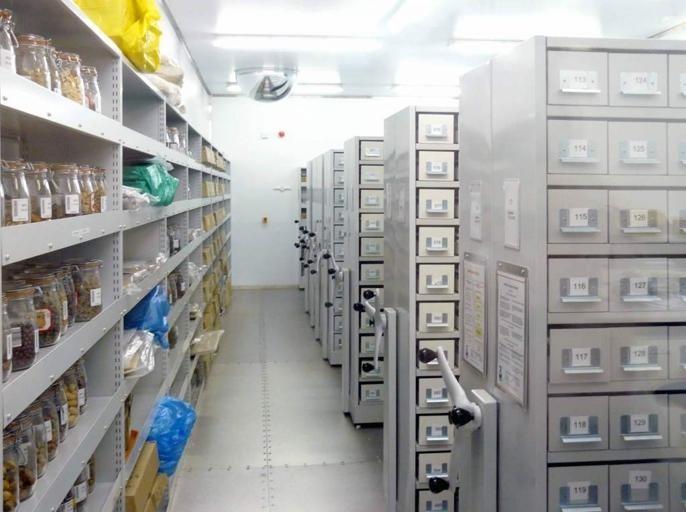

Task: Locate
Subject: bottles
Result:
[63,369,78,429]
[55,381,68,443]
[8,288,40,371]
[80,162,95,216]
[2,421,18,512]
[72,52,86,108]
[93,167,108,214]
[54,165,81,220]
[0,9,19,75]
[61,257,104,337]
[59,488,76,512]
[83,64,104,114]
[29,407,49,479]
[58,53,73,100]
[190,302,201,321]
[35,35,51,89]
[2,298,14,383]
[6,159,31,226]
[76,359,87,415]
[166,126,187,152]
[167,268,186,305]
[29,278,61,348]
[48,44,60,95]
[72,473,88,510]
[12,415,37,502]
[19,34,38,83]
[165,222,184,259]
[43,395,58,462]
[29,162,53,223]
[0,159,6,228]
[83,455,95,495]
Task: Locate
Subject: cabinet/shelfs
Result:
[420,36,686,511]
[353,107,459,509]
[0,0,231,512]
[293,148,348,364]
[328,137,386,428]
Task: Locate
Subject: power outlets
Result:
[262,216,268,224]
